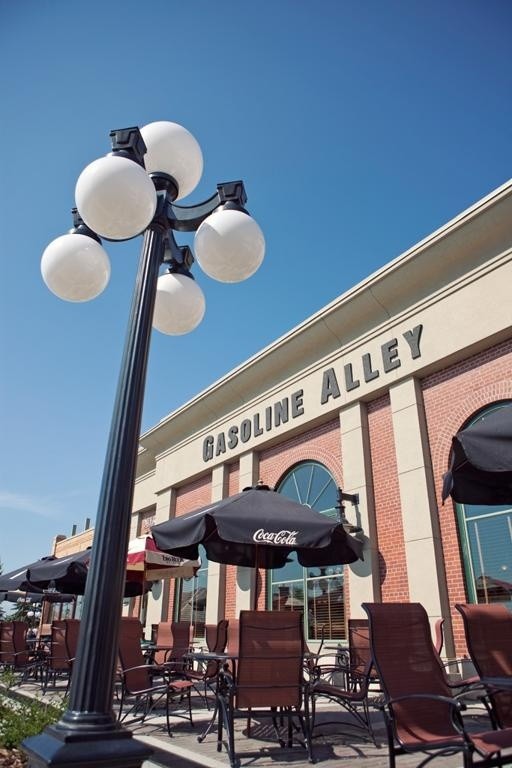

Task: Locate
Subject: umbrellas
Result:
[149,479,366,610]
[0,527,201,619]
[440,407,512,506]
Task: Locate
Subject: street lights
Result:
[19,119,265,767]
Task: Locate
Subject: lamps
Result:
[335,487,364,538]
